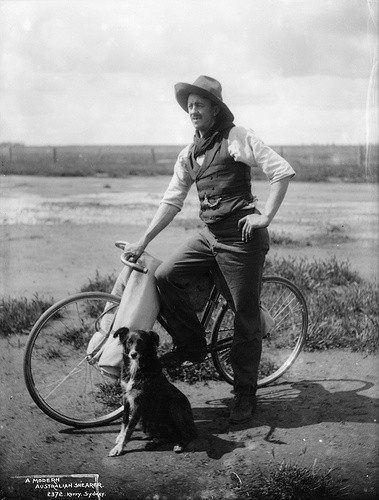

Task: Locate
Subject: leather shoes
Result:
[158,345,207,366]
[229,393,256,423]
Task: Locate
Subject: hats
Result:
[174,76,234,125]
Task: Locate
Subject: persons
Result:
[124,76,297,424]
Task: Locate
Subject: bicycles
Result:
[23,240,309,428]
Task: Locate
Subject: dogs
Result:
[108,327,196,458]
